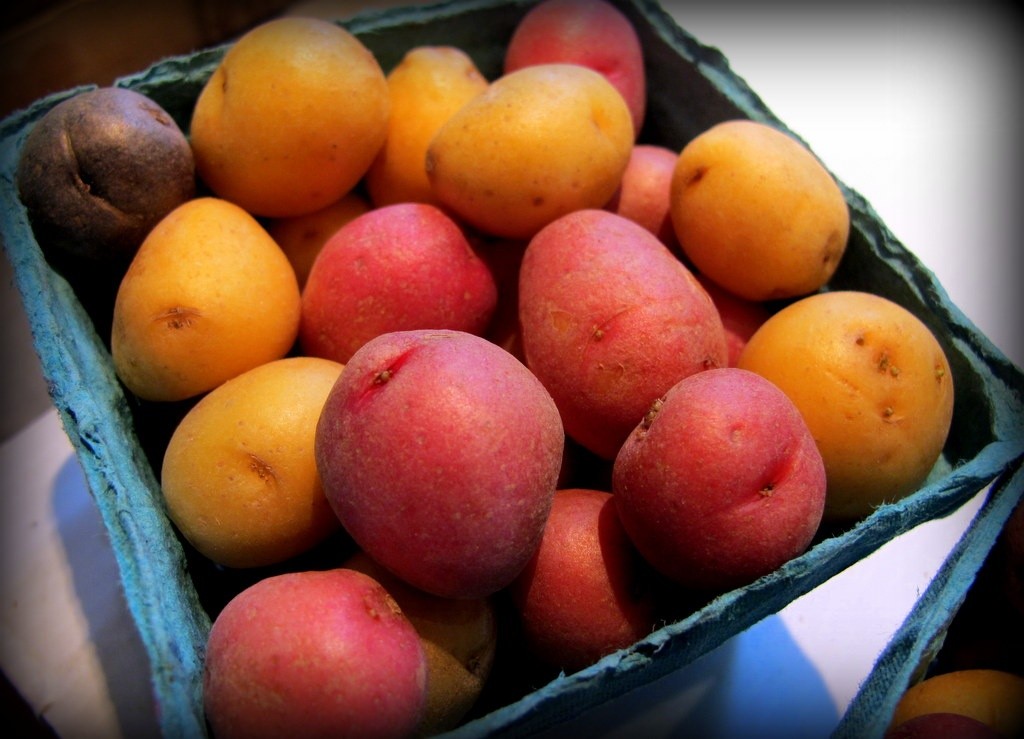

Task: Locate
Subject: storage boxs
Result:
[0,0,1024,739]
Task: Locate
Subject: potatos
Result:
[885,668,1024,739]
[18,0,954,739]
